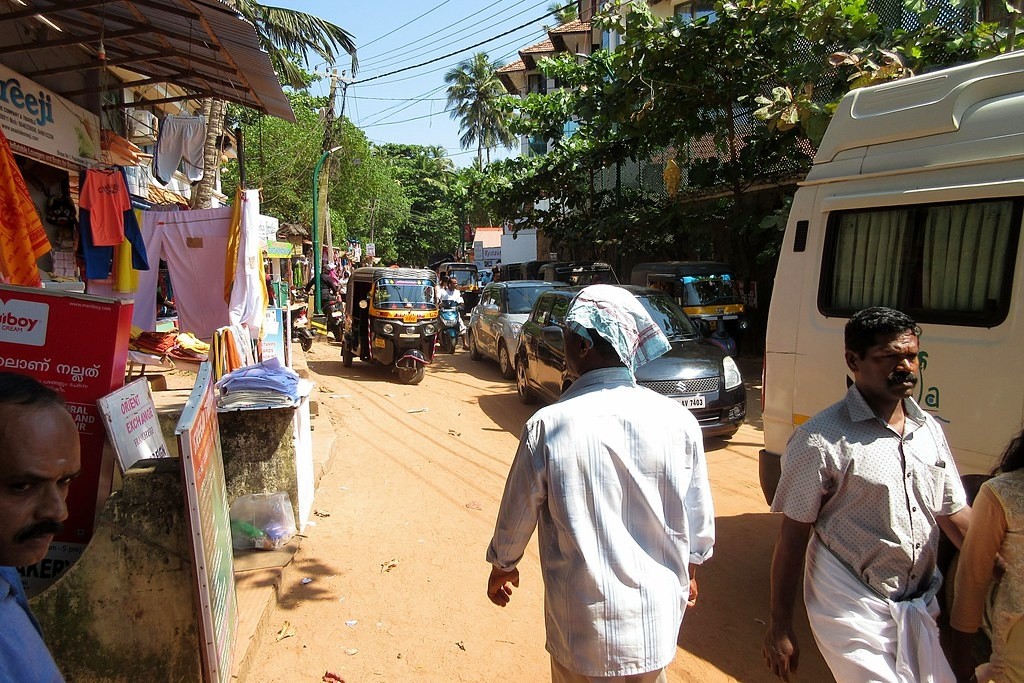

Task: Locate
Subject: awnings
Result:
[0,0,298,124]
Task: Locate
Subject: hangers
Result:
[158,190,172,206]
[167,99,205,121]
[83,157,125,179]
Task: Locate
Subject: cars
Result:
[466,279,573,384]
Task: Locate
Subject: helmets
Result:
[323,264,333,275]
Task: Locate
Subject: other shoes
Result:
[463,345,470,350]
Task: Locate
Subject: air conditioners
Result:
[127,109,159,142]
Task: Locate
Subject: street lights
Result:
[311,145,343,317]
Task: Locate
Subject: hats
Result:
[486,269,492,273]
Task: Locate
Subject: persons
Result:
[303,263,351,317]
[156,270,178,318]
[648,280,674,299]
[435,271,469,349]
[510,289,533,309]
[950,427,1024,683]
[469,260,502,292]
[0,370,81,683]
[763,306,973,683]
[486,284,715,683]
[587,271,603,284]
[383,286,405,308]
[264,264,277,307]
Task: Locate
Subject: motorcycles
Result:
[514,284,748,442]
[630,261,750,359]
[337,269,444,385]
[496,260,624,297]
[439,262,484,318]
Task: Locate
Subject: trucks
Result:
[757,49,1023,547]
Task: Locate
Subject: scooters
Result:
[282,306,317,352]
[435,299,463,355]
[324,295,348,342]
